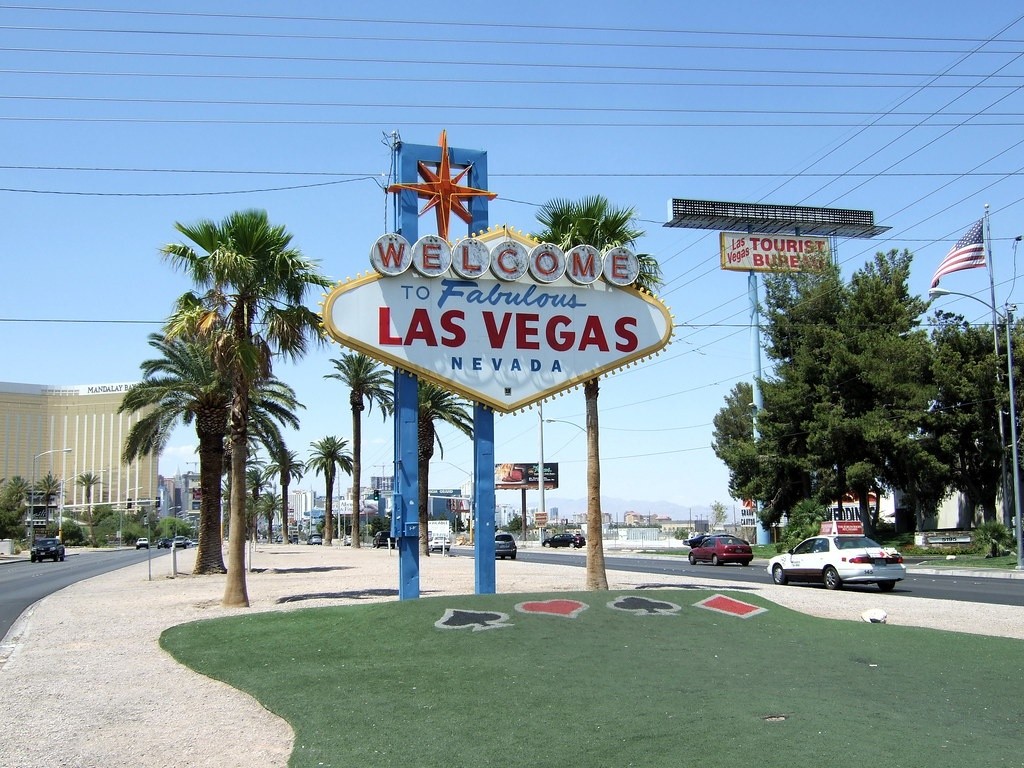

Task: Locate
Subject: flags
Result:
[932,216,988,288]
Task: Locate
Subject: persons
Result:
[575,534,582,549]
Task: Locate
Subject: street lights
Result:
[928,287,1024,570]
[430,460,474,545]
[120,486,144,549]
[30,448,72,552]
[59,470,107,544]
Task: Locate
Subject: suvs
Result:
[494,533,517,559]
[542,533,586,548]
[372,531,396,549]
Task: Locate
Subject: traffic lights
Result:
[373,489,380,500]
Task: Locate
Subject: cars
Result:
[275,535,283,542]
[683,533,754,566]
[428,536,451,551]
[135,537,148,550]
[157,535,199,549]
[684,534,711,549]
[766,531,906,591]
[30,538,65,562]
[343,536,352,546]
[306,536,322,545]
